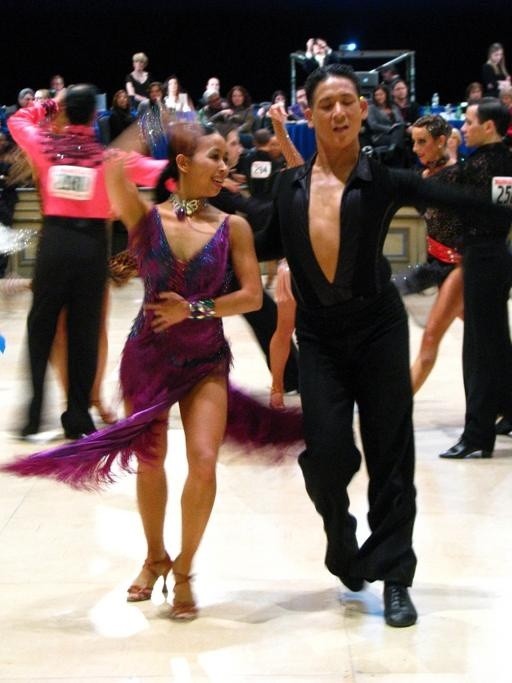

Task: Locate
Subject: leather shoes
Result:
[496,418,512,434]
[440,440,493,458]
[340,565,363,592]
[384,581,417,627]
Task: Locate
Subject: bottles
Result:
[431,91,440,111]
[444,102,464,119]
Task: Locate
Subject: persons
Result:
[0,118,311,621]
[0,40,512,461]
[229,62,477,628]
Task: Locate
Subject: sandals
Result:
[90,397,118,424]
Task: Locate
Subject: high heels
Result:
[172,557,198,621]
[127,550,172,602]
